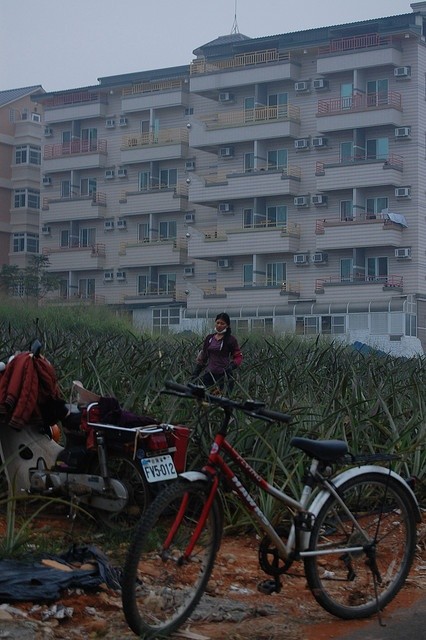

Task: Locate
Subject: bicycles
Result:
[121,381,422,637]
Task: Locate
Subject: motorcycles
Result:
[0,339,178,533]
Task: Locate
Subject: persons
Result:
[188,312,244,402]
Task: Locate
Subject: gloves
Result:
[187,364,201,383]
[221,363,237,377]
